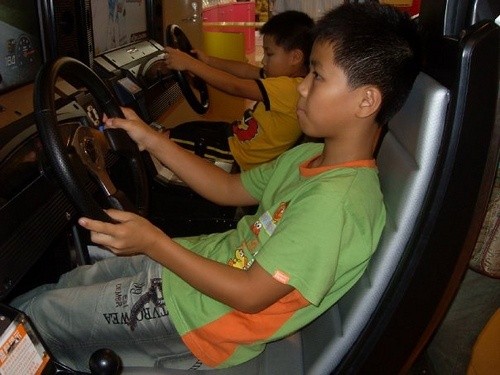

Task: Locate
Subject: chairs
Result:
[121,19,500,375]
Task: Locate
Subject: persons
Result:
[3,0,419,375]
[129,10,316,188]
